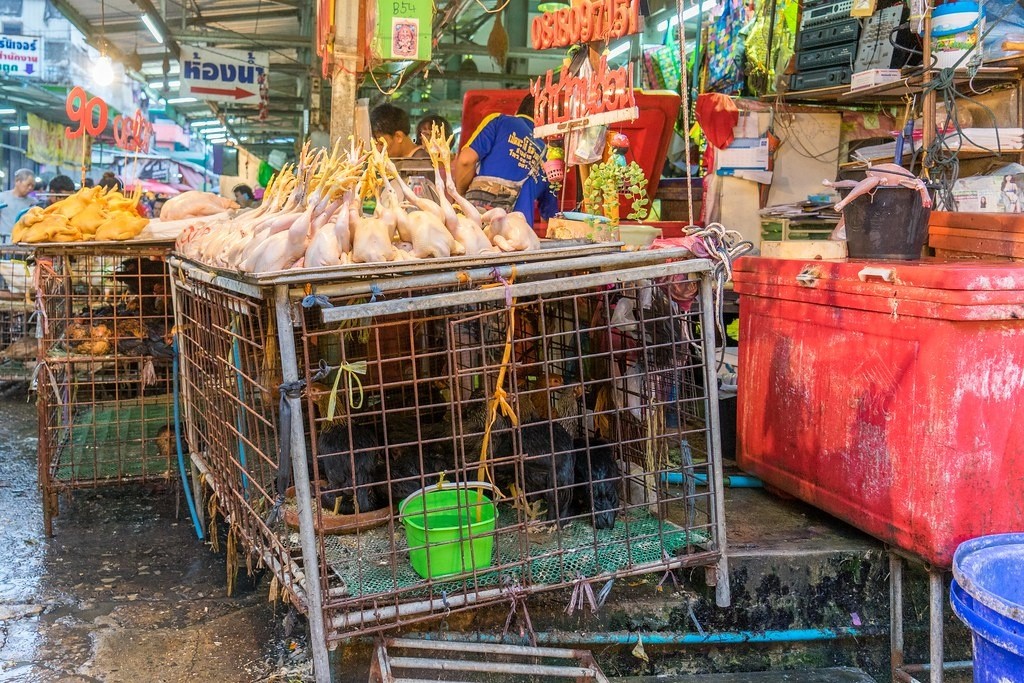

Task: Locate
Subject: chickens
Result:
[11,185,209,454]
[264,373,621,530]
[157,120,644,275]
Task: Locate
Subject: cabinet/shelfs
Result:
[760,52,1024,209]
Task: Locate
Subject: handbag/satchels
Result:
[463,175,522,214]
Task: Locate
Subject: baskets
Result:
[542,159,564,184]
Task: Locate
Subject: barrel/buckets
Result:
[949,532,1024,682]
[397,481,501,579]
[931,1,985,69]
[837,168,936,260]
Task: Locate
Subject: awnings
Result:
[179,166,205,190]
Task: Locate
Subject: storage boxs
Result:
[851,69,901,91]
[732,255,1024,569]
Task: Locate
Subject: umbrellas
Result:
[129,179,179,193]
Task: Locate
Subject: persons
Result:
[0,169,37,244]
[81,178,94,188]
[415,114,455,151]
[980,196,987,208]
[37,175,74,208]
[1001,175,1021,212]
[371,104,435,189]
[234,185,260,208]
[100,172,120,192]
[154,198,167,217]
[449,90,559,418]
